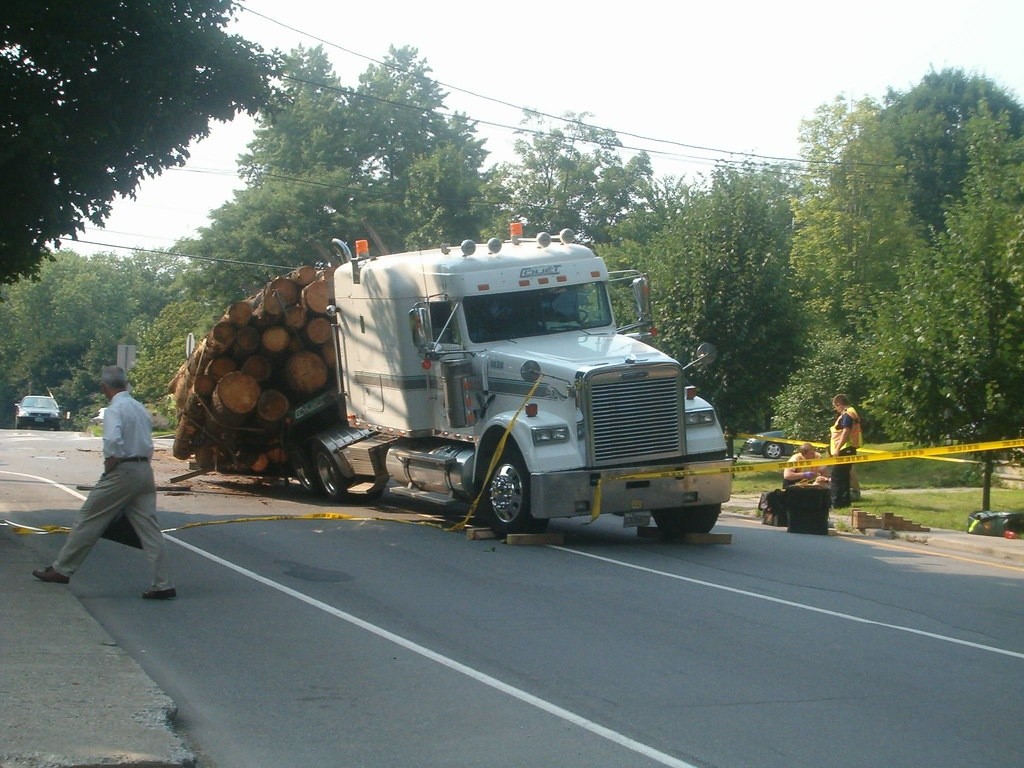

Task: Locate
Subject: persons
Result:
[784,394,862,510]
[33,367,178,598]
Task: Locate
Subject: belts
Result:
[121,457,148,462]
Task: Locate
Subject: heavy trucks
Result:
[214,221,735,544]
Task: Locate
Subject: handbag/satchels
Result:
[966,510,1024,537]
[100,513,143,550]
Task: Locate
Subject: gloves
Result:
[803,472,816,480]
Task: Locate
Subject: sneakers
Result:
[32,566,69,584]
[143,588,177,601]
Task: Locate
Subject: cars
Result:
[15,396,61,432]
[88,407,108,427]
[746,430,793,460]
